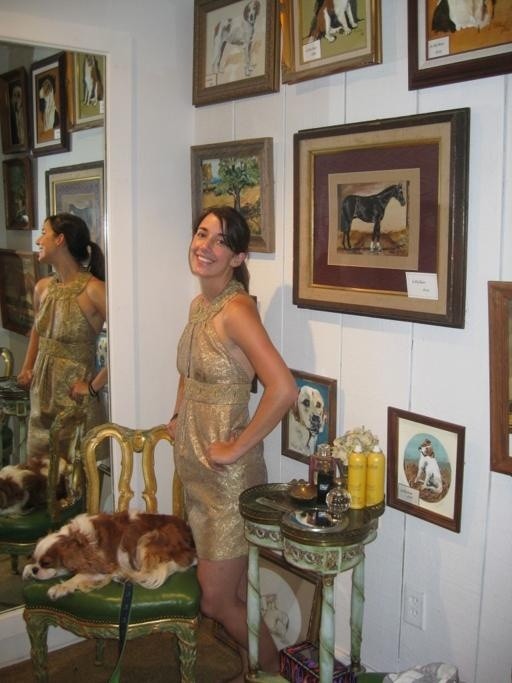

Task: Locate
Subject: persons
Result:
[17,213,106,499]
[168,205,297,674]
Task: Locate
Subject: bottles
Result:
[308,442,385,509]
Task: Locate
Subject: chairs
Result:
[1,402,103,557]
[20,421,204,682]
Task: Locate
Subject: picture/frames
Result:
[406,0,512,93]
[278,0,384,85]
[189,136,275,255]
[1,49,106,343]
[192,0,279,107]
[385,404,466,536]
[292,105,470,331]
[487,278,512,480]
[280,365,339,475]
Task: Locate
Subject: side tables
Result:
[236,479,386,682]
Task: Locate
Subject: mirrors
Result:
[0,36,119,672]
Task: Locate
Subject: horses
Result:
[340,182,406,252]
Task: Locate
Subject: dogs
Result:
[39,79,59,131]
[83,55,103,106]
[22,509,199,601]
[289,385,324,456]
[0,448,85,519]
[301,0,358,43]
[12,86,28,147]
[211,0,260,76]
[431,0,493,31]
[414,438,443,494]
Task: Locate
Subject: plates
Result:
[295,510,323,528]
